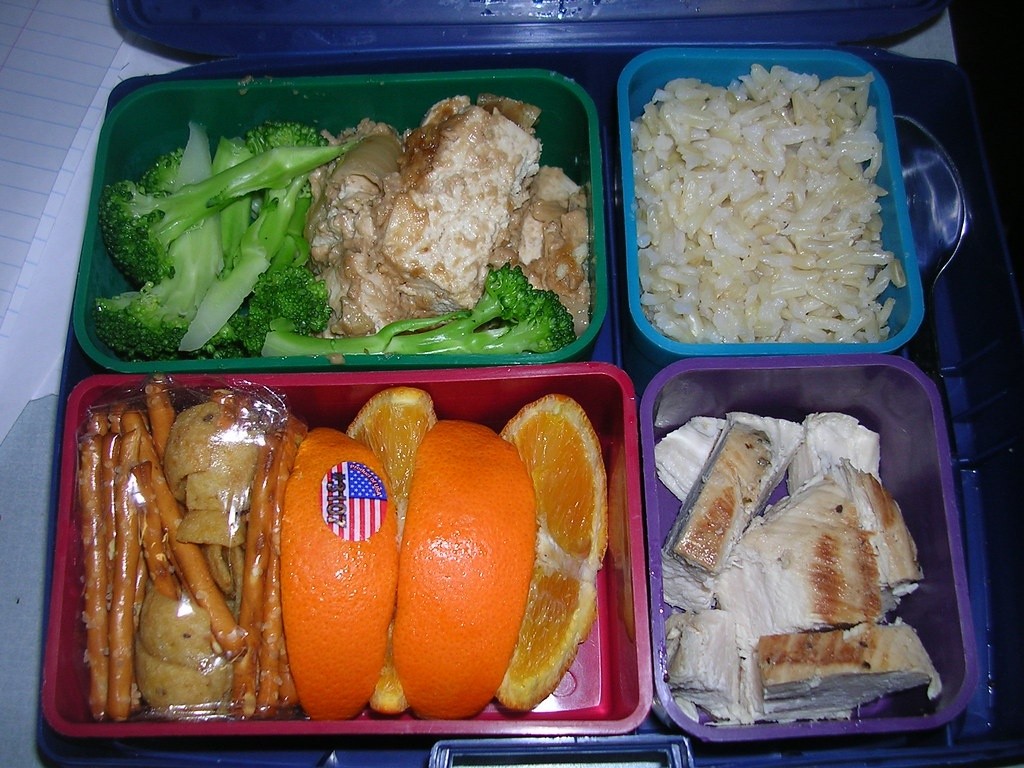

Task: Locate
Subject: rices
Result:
[629,64,908,344]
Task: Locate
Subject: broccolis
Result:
[89,118,576,362]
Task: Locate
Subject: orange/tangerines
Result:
[277,388,609,722]
